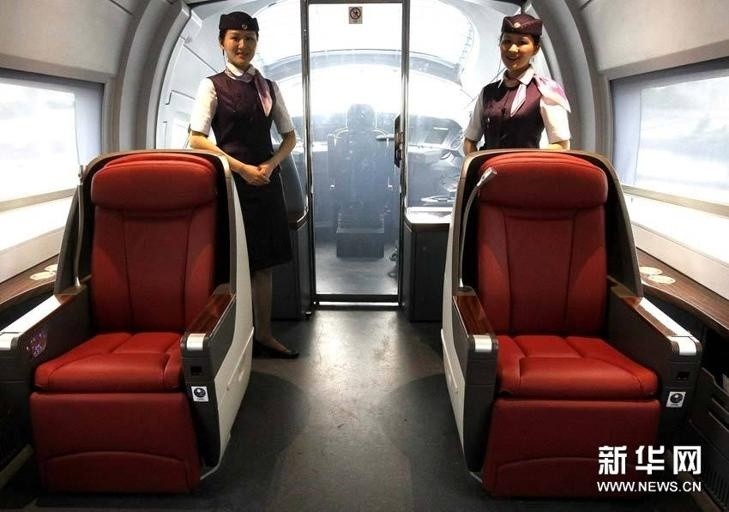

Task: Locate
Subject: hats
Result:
[219,11,259,31]
[501,13,543,35]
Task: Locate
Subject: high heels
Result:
[253,335,300,360]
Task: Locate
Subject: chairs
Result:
[439,142,701,501]
[11,140,260,494]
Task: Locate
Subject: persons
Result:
[463,14,571,156]
[188,12,299,359]
